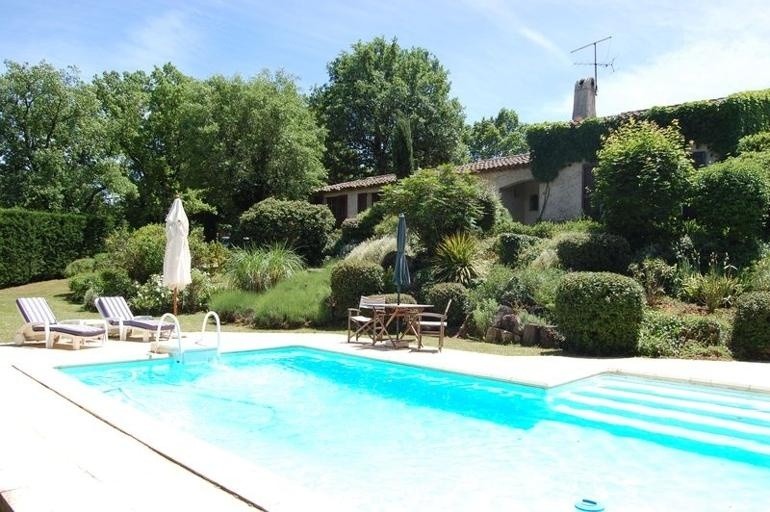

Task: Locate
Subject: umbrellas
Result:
[391,212,413,340]
[161,194,194,316]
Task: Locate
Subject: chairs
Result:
[93,296,177,345]
[346,296,385,345]
[412,298,453,353]
[16,296,108,352]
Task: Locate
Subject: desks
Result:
[366,303,434,350]
